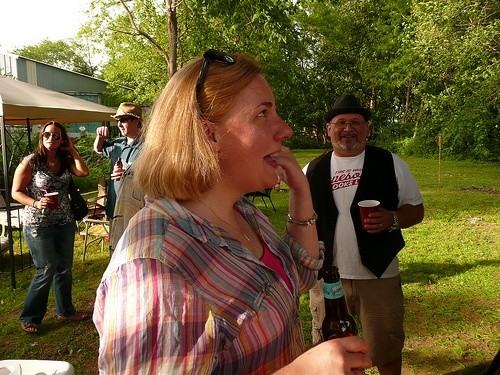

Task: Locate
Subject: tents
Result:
[0,75,119,290]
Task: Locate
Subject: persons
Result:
[93,49,373,375]
[11,121,89,331]
[300,92,425,375]
[94,102,143,218]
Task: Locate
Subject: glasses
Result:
[329,120,363,128]
[42,132,60,140]
[196,48,235,97]
[116,118,131,124]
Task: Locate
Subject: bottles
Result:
[321,266,365,375]
[114,157,122,182]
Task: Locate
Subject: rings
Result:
[380,224,382,229]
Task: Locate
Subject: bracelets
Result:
[33,200,38,208]
[287,210,318,226]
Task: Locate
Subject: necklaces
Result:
[48,160,58,166]
[200,200,250,241]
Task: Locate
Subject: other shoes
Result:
[21,322,41,333]
[58,311,88,320]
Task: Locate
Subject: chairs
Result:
[244,188,277,212]
[78,215,110,262]
[85,176,108,214]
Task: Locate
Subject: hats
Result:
[324,94,372,122]
[110,102,143,121]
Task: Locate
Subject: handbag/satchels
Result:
[68,190,89,221]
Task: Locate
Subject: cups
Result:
[44,192,59,210]
[358,200,380,230]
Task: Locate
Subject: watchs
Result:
[388,210,399,232]
